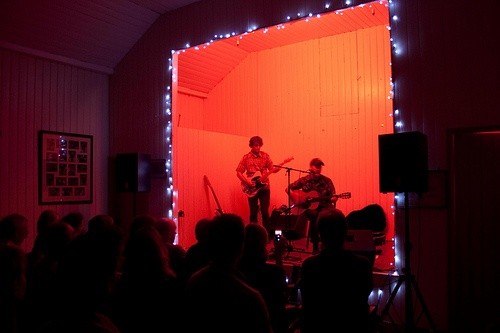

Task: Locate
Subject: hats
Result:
[310,158,324,167]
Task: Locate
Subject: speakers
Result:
[115,152,151,193]
[378,131,429,195]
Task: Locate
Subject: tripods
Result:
[375,192,438,333]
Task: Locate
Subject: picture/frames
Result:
[38,129,94,205]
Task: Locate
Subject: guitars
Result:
[288,190,351,215]
[240,157,294,198]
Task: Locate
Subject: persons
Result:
[300,208,373,333]
[236,136,282,231]
[285,158,337,250]
[0,211,274,333]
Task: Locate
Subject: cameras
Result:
[275,230,282,242]
